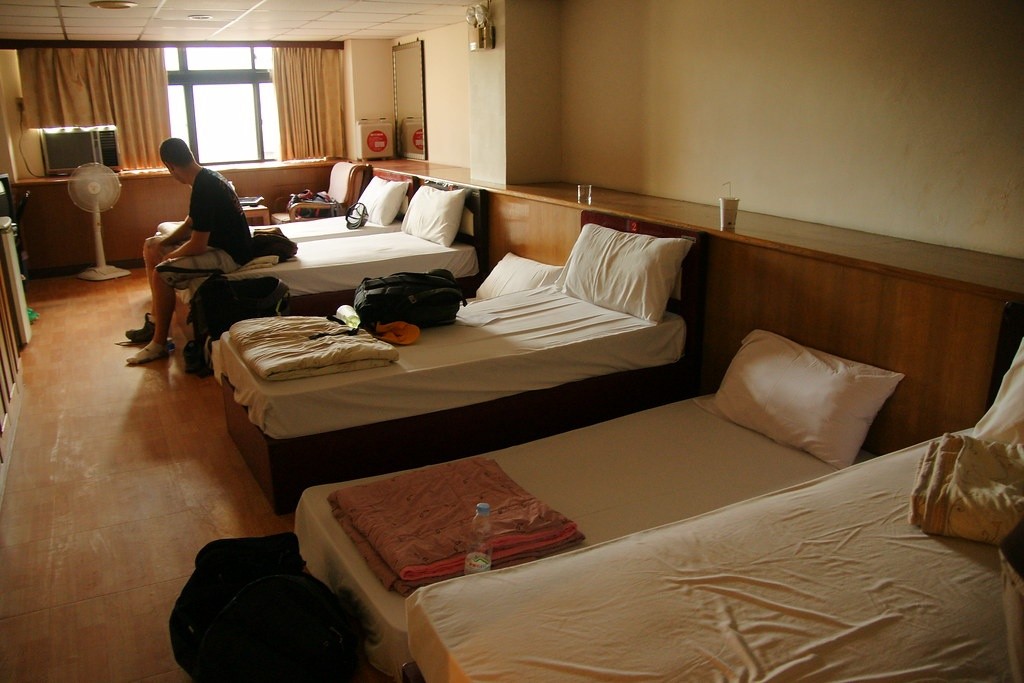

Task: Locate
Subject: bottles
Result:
[336,305,361,328]
[464,503,493,576]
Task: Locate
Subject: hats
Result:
[345,202,369,230]
[374,321,420,345]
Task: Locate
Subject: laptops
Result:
[239,196,262,206]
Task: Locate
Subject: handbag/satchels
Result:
[252,227,297,260]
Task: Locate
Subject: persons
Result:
[125,137,253,365]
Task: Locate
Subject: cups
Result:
[719,197,739,229]
[577,184,591,204]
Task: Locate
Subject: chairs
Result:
[270,161,373,227]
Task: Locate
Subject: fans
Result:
[67,162,132,282]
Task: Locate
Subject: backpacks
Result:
[355,268,468,330]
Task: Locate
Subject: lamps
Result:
[465,3,496,51]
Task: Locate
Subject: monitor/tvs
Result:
[39,126,122,176]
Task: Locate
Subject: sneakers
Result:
[126,312,154,341]
[183,340,197,373]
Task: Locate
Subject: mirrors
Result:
[391,40,429,161]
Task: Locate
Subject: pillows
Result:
[350,174,410,227]
[553,224,695,324]
[474,252,564,300]
[970,338,1024,447]
[401,184,467,246]
[692,329,905,472]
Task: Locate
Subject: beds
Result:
[406,339,1024,683]
[294,395,876,682]
[156,217,686,518]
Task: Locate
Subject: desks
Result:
[241,202,270,226]
